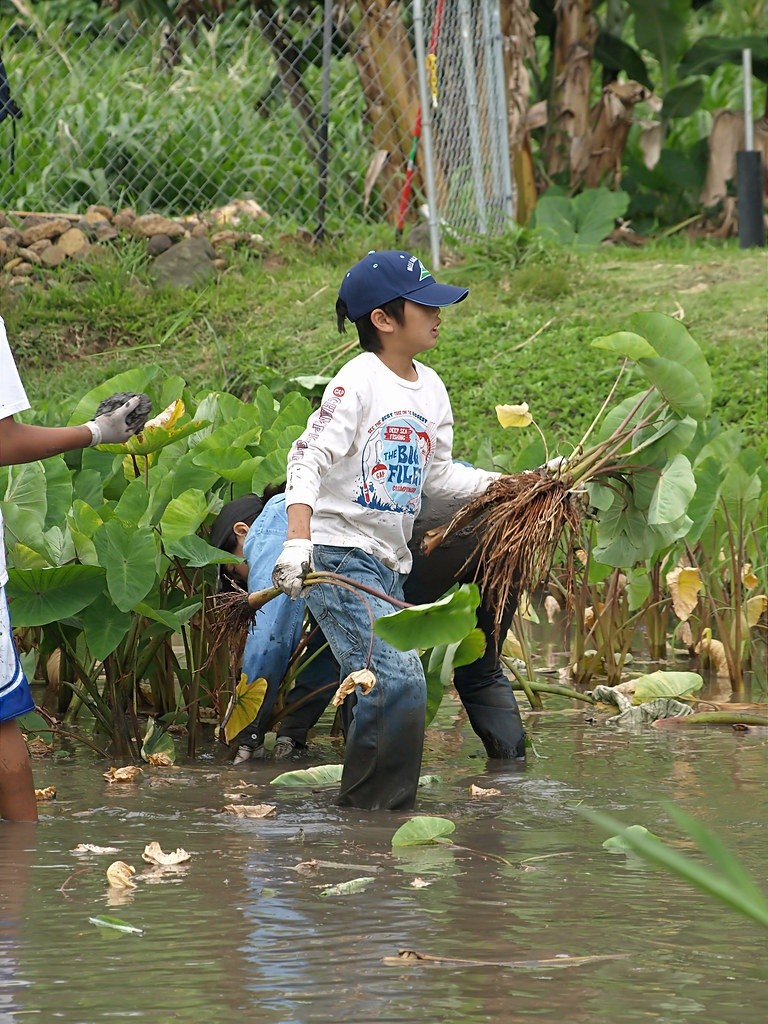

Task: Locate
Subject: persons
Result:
[209,494,526,764]
[272,250,585,814]
[0,318,147,820]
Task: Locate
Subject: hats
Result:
[210,494,264,591]
[338,250,468,325]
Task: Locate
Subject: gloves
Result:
[84,397,140,448]
[523,456,584,500]
[272,736,296,760]
[271,539,313,601]
[232,743,265,765]
